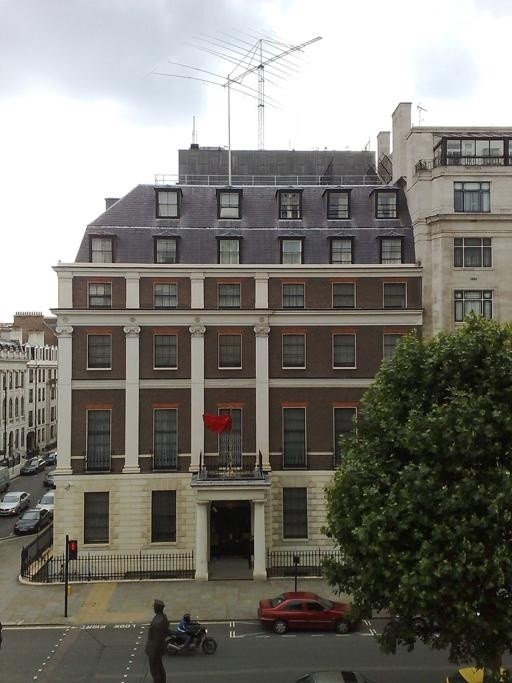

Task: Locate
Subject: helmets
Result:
[184,614,192,621]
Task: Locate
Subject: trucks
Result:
[0,466,10,492]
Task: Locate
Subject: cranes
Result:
[223,36,323,150]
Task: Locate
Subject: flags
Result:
[203,411,231,435]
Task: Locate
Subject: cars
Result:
[445,668,512,683]
[258,592,361,633]
[294,671,372,683]
[20,451,57,476]
[0,490,54,535]
[395,605,480,633]
[43,471,54,485]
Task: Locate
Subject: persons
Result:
[145,599,169,683]
[176,614,201,649]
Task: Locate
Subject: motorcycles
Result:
[165,616,218,655]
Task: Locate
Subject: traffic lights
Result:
[69,540,78,560]
